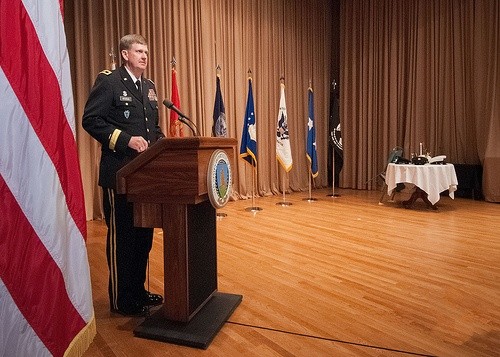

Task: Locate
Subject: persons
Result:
[82,34,167,316]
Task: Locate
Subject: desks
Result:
[386,163,459,210]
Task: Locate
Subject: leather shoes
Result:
[144,293,163,306]
[112,304,148,317]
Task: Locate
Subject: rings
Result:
[138,146,142,149]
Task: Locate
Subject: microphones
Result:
[163,99,185,117]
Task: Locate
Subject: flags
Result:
[0,0,97,357]
[276,83,293,172]
[327,88,343,179]
[305,85,320,177]
[168,68,183,138]
[240,76,258,171]
[211,75,228,137]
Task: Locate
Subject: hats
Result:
[395,155,447,165]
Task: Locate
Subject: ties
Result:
[136,80,142,95]
